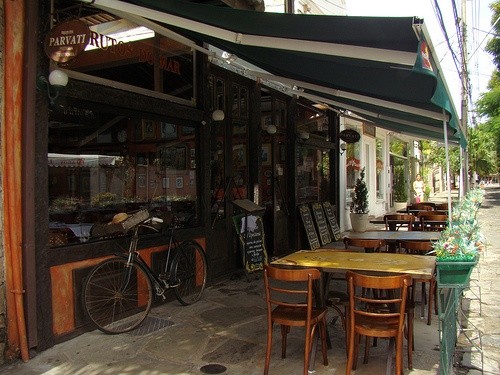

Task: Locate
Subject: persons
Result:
[413,174,426,203]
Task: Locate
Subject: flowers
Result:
[427,185,488,261]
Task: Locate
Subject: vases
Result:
[436,254,479,288]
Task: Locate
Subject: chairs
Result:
[264,202,455,374]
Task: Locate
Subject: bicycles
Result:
[81,212,209,335]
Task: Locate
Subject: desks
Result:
[370,214,446,228]
[396,209,448,215]
[343,231,441,325]
[270,250,437,370]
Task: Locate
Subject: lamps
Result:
[336,143,347,156]
[40,65,69,100]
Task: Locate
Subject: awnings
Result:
[84,0,469,149]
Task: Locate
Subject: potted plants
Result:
[350,166,370,231]
